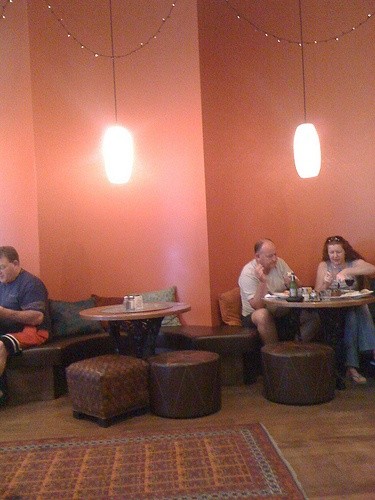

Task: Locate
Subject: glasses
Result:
[326,236,342,243]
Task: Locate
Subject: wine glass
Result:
[345,274,355,293]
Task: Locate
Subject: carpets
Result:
[0,421,309,500]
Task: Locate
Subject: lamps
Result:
[293,0,322,178]
[103,0,137,183]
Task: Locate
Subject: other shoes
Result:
[0,391,8,404]
[346,370,367,385]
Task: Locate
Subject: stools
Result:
[260,340,337,406]
[66,356,150,426]
[153,349,222,418]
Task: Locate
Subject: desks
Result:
[264,291,375,389]
[77,301,191,360]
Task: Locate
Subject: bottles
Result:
[297,288,317,301]
[289,272,297,298]
[122,294,143,310]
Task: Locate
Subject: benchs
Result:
[4,321,254,406]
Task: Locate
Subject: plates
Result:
[274,291,289,299]
[340,291,374,299]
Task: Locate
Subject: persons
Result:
[238,238,321,346]
[0,246,50,399]
[316,236,375,385]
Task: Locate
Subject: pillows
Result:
[218,288,245,328]
[89,294,137,330]
[129,287,184,329]
[47,297,105,338]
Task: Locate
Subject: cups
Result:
[319,290,331,302]
